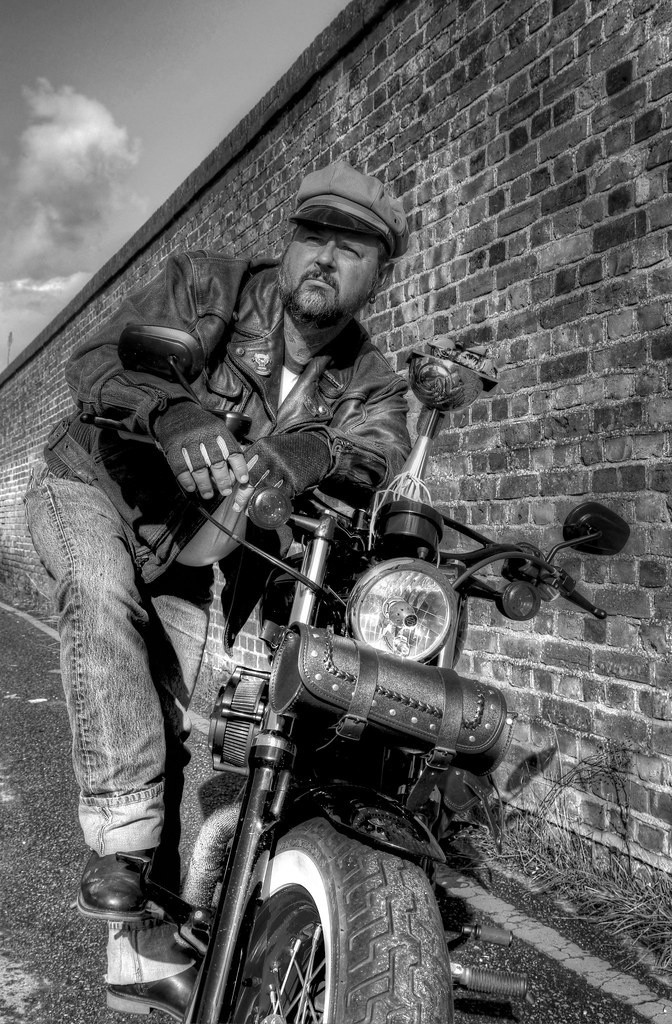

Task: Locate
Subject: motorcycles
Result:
[78,316,632,1024]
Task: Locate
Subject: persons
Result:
[19,166,416,1022]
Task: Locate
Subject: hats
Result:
[287,161,409,263]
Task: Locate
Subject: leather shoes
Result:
[107,926,194,1016]
[79,847,155,923]
